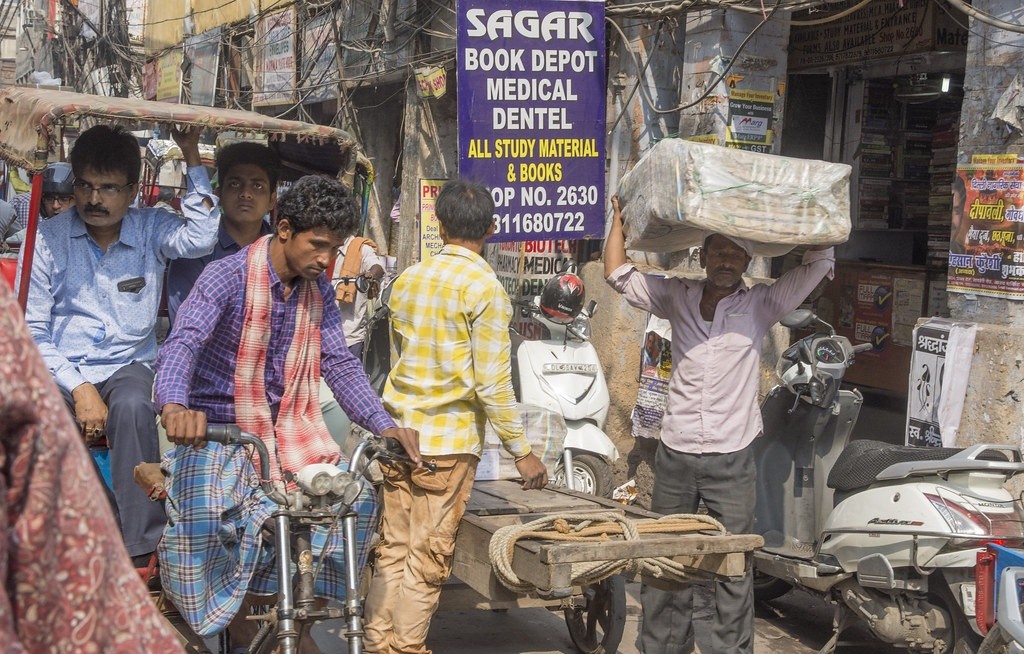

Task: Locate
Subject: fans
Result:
[893,74,942,105]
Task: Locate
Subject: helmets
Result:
[40,162,74,194]
[540,272,585,325]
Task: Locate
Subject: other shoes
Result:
[133,552,163,592]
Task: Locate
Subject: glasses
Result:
[72,182,134,193]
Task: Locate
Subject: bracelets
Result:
[515,452,532,463]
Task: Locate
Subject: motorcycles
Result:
[1,87,438,653]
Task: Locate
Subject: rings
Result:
[85,428,102,436]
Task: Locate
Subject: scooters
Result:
[480,261,620,500]
[751,309,1024,654]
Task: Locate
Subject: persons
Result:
[643,331,656,366]
[324,231,387,366]
[166,142,279,330]
[151,174,422,654]
[949,174,966,254]
[12,124,223,589]
[0,163,76,259]
[604,194,835,654]
[363,183,549,654]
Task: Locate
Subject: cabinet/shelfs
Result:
[855,78,932,232]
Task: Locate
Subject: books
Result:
[857,78,932,229]
[929,109,958,267]
